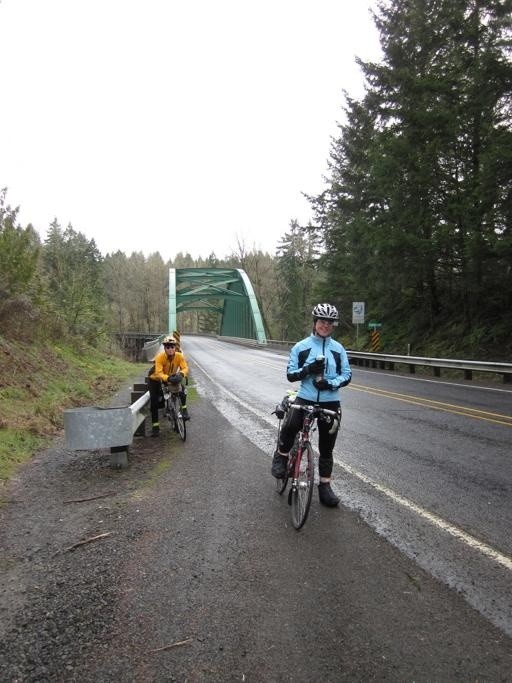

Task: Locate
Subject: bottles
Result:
[316,355,325,383]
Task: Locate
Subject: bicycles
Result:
[271,396,341,530]
[161,373,188,441]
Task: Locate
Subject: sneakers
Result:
[152,425,160,436]
[271,450,289,479]
[182,407,190,420]
[318,483,340,507]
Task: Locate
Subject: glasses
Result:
[167,346,174,349]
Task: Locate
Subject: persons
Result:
[148,336,190,437]
[271,303,352,507]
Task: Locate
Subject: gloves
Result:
[168,373,183,383]
[309,358,326,374]
[312,377,330,390]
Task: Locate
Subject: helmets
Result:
[162,336,177,344]
[312,303,340,320]
